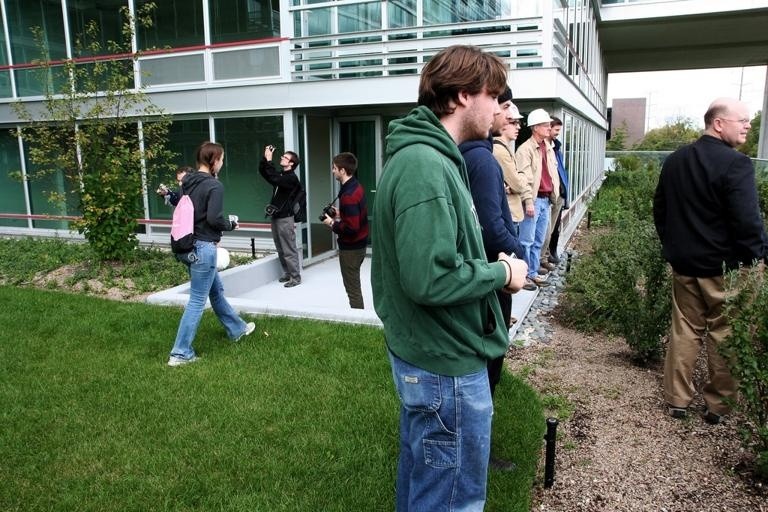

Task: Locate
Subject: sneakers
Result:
[667,403,687,418]
[702,410,722,424]
[236,322,256,343]
[279,273,290,282]
[523,252,560,291]
[284,280,300,287]
[167,355,202,366]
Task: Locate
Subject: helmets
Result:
[508,102,524,120]
[527,108,555,127]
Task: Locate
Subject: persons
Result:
[324,151,370,309]
[457,83,526,428]
[490,103,529,332]
[651,94,766,429]
[514,107,570,295]
[155,166,195,208]
[499,258,512,288]
[259,144,302,287]
[369,45,529,512]
[168,140,256,369]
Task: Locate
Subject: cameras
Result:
[268,145,273,152]
[318,206,336,222]
[228,214,239,229]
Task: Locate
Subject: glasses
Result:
[509,120,521,126]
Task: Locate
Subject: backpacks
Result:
[170,182,201,254]
